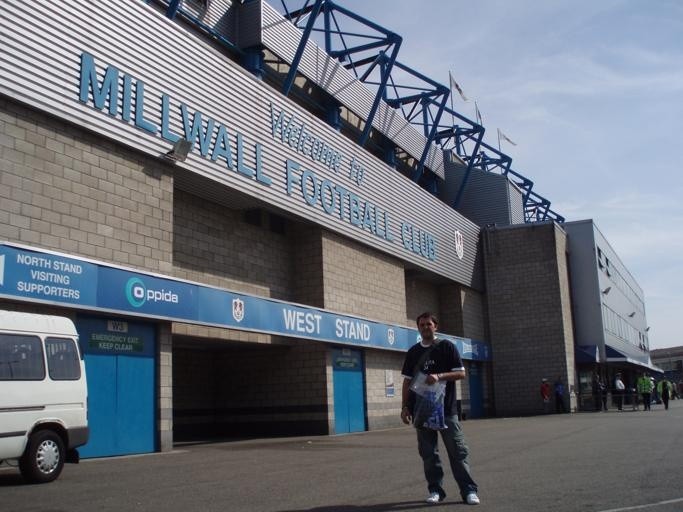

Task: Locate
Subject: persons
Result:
[541,375,566,414]
[592,370,683,412]
[401,311,480,505]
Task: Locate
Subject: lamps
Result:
[165,138,192,162]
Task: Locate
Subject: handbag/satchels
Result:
[408,371,449,430]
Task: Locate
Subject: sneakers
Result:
[426,490,442,503]
[466,491,481,504]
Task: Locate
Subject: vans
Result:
[1,308,89,484]
